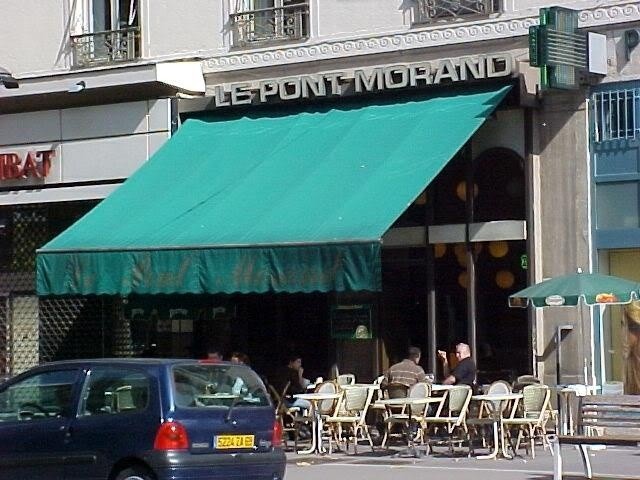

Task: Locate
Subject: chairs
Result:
[258,358,564,462]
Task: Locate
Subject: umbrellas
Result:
[508,268,640,424]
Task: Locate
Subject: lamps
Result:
[65,79,88,97]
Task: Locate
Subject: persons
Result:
[437,343,476,458]
[198,342,225,364]
[280,356,326,452]
[53,385,72,420]
[241,336,273,369]
[380,348,433,445]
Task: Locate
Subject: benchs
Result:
[544,389,640,480]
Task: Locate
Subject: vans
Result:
[0,355,288,480]
